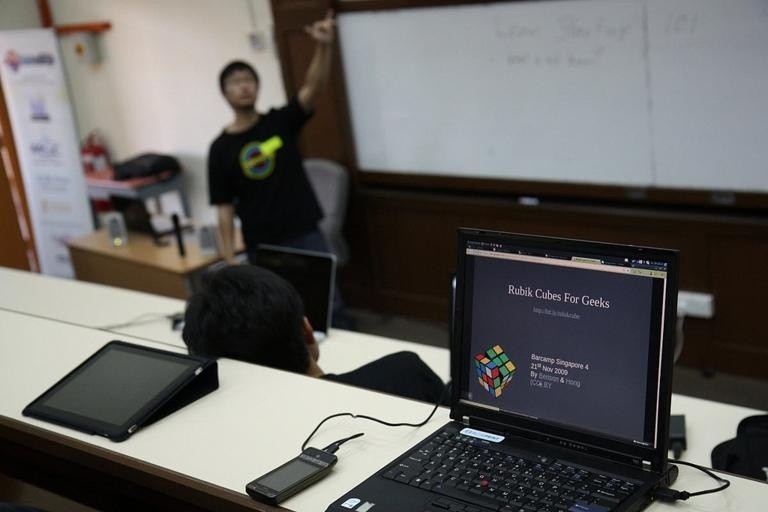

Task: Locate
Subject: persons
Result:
[204,7,344,333]
[182,264,452,408]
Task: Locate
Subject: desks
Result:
[66,214,247,299]
[85,165,188,230]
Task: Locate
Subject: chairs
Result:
[302,157,348,266]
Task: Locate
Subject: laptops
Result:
[324,228,680,512]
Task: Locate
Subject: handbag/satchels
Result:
[115,154,181,180]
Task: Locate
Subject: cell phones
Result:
[246,447,338,505]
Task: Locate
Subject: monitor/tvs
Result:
[22,340,219,443]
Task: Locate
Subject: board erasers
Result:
[519,197,539,203]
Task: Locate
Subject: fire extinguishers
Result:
[79,128,115,214]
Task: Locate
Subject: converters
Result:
[668,415,687,450]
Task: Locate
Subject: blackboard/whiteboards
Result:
[336,0,768,224]
